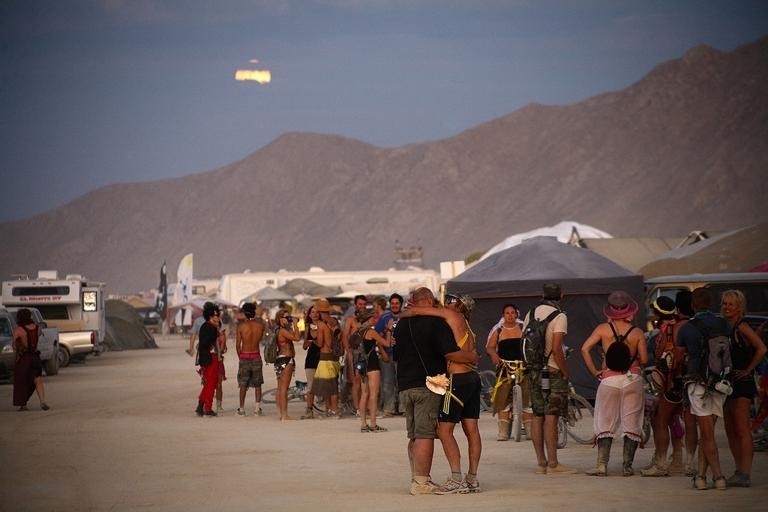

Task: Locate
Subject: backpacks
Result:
[325,319,344,357]
[520,305,560,369]
[689,315,734,397]
[606,322,638,371]
[264,327,280,362]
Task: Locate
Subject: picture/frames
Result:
[83,291,97,312]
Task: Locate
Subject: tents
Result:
[102,298,160,351]
[446,235,646,411]
[127,276,356,340]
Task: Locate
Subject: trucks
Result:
[1,270,105,350]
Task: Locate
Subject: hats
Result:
[603,290,678,320]
[445,287,475,311]
[317,298,332,311]
[543,282,561,298]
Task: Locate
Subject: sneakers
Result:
[195,406,269,416]
[410,478,480,495]
[277,406,359,420]
[40,401,49,411]
[640,460,752,490]
[536,464,578,474]
[361,425,386,432]
[17,406,28,411]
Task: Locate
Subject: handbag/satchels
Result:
[425,374,450,396]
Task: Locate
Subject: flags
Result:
[154,262,168,319]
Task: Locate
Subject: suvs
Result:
[137,307,163,328]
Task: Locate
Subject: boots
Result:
[586,437,612,475]
[497,419,532,440]
[623,434,638,476]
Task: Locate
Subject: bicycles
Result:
[259,366,497,420]
[506,361,526,440]
[552,346,596,449]
[641,382,654,444]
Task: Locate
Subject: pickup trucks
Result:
[1,307,99,376]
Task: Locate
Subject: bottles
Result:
[541,366,551,392]
[715,380,734,396]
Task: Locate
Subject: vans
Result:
[646,274,766,336]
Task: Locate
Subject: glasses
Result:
[444,297,456,303]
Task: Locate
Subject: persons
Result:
[187,290,404,431]
[581,287,767,490]
[9,308,50,411]
[486,283,578,475]
[392,287,482,495]
